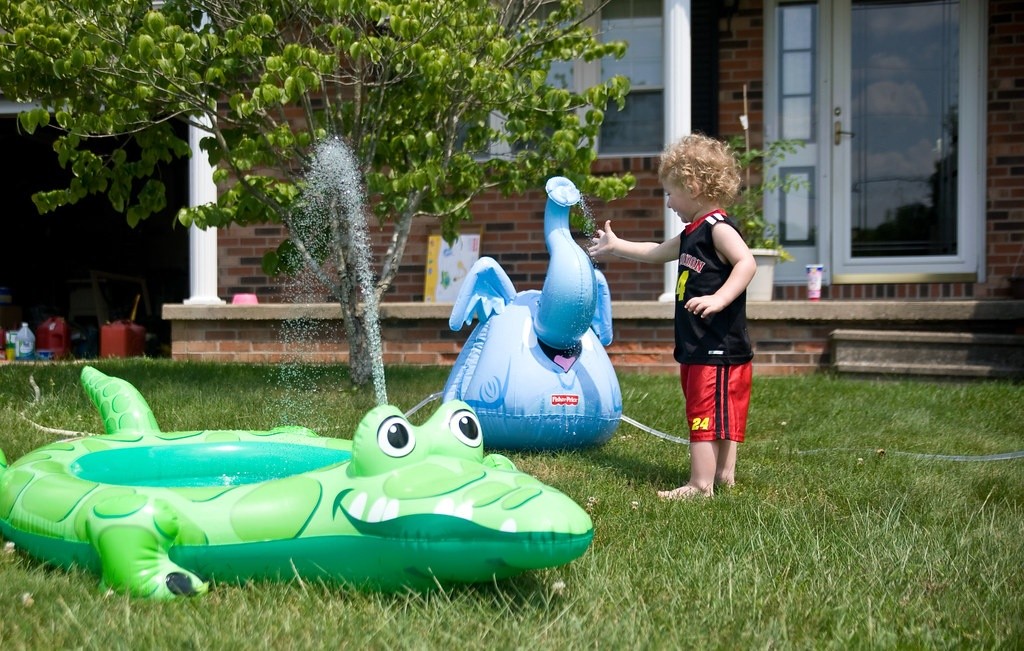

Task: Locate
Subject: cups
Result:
[805,264,823,301]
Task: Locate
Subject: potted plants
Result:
[724,134,813,301]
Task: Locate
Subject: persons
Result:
[588,133,758,501]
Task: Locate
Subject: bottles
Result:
[16,322,36,361]
[101,319,147,359]
[32,317,69,360]
